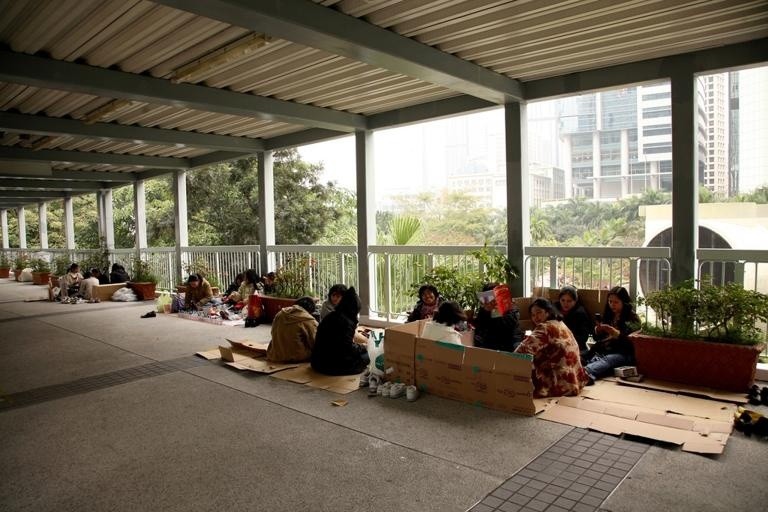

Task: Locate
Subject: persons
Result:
[405,282,524,353]
[184,273,221,311]
[580,287,642,384]
[220,269,287,321]
[56,263,130,300]
[552,286,593,352]
[513,298,589,398]
[320,284,368,344]
[266,297,319,363]
[311,286,370,375]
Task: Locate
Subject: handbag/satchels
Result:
[171,296,185,312]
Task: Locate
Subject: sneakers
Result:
[369,374,382,392]
[390,383,407,398]
[406,385,420,401]
[59,296,79,304]
[141,311,156,318]
[85,297,100,303]
[377,380,393,396]
[359,371,376,387]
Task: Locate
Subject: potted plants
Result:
[0,235,159,299]
[258,253,318,321]
[627,274,768,394]
[401,240,521,329]
[175,257,224,296]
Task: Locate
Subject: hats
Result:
[439,301,468,320]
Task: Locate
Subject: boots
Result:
[246,316,259,327]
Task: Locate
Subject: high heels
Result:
[734,406,768,437]
